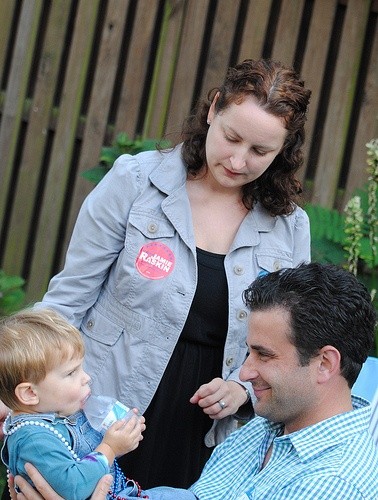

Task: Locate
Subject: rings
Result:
[218,400,227,410]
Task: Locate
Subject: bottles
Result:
[83,396,132,436]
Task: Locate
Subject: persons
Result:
[6,263,378,500]
[28,58,314,490]
[0,309,198,500]
